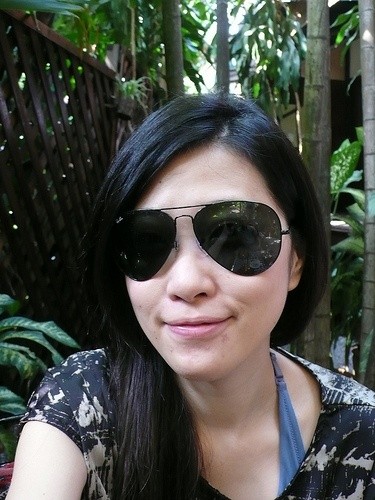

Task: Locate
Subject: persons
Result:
[5,89,375,500]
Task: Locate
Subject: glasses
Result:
[108,202,294,283]
